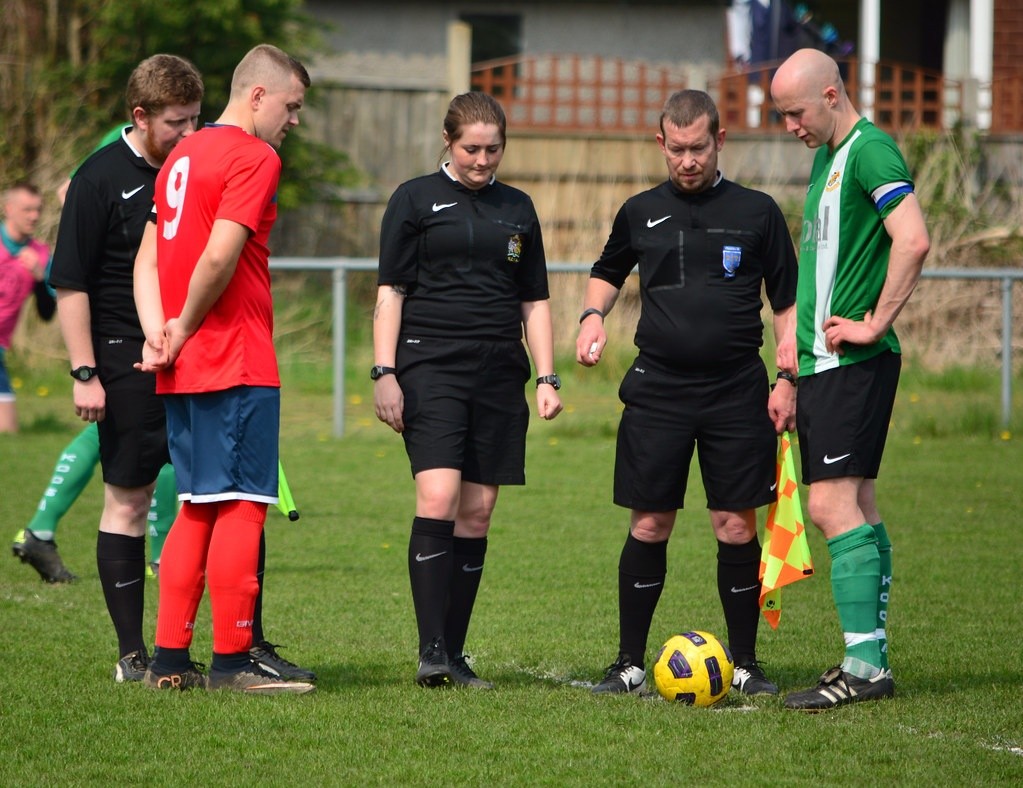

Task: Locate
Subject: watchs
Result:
[371,366,397,380]
[70,366,97,382]
[536,373,561,390]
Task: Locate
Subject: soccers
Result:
[654,631,735,707]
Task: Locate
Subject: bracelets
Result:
[777,372,797,386]
[580,308,605,324]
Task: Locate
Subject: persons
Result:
[771,48,931,713]
[133,45,318,696]
[14,111,183,585]
[0,181,57,435]
[370,91,563,691]
[48,54,301,685]
[576,90,797,698]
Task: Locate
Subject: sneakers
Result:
[114,649,152,680]
[417,637,455,687]
[783,663,896,712]
[208,660,316,698]
[144,662,210,690]
[449,654,493,688]
[12,528,79,582]
[249,641,316,684]
[590,653,646,693]
[732,657,778,699]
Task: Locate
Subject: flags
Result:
[758,432,814,630]
[275,459,297,516]
